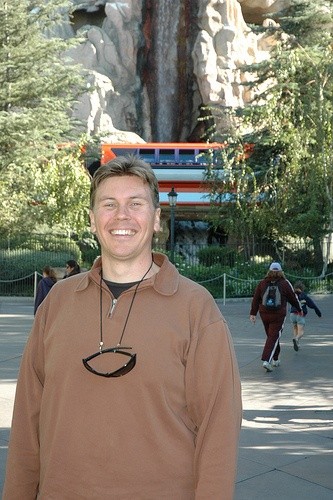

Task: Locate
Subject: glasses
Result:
[82,347,136,378]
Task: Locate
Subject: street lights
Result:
[166,185,178,265]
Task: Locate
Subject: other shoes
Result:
[263,361,273,371]
[293,338,299,351]
[273,360,280,367]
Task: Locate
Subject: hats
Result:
[269,263,282,271]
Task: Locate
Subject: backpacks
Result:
[262,277,281,311]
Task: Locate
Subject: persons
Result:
[288,283,323,352]
[34,266,57,317]
[2,154,242,500]
[250,262,303,372]
[63,260,81,279]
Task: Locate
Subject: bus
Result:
[57,140,284,205]
